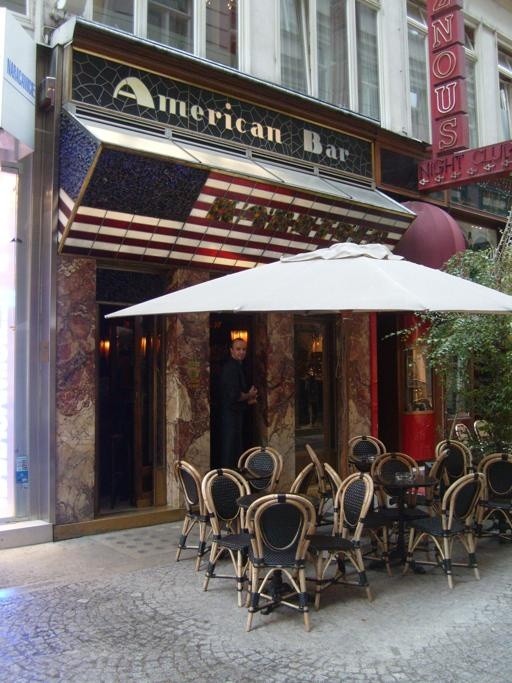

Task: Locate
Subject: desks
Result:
[237,494,320,593]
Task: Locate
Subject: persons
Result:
[214,337,259,470]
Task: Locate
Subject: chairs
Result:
[201,469,250,608]
[172,442,332,572]
[324,435,488,590]
[292,472,375,610]
[454,419,512,545]
[244,494,317,632]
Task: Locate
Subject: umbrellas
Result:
[104,239,512,482]
